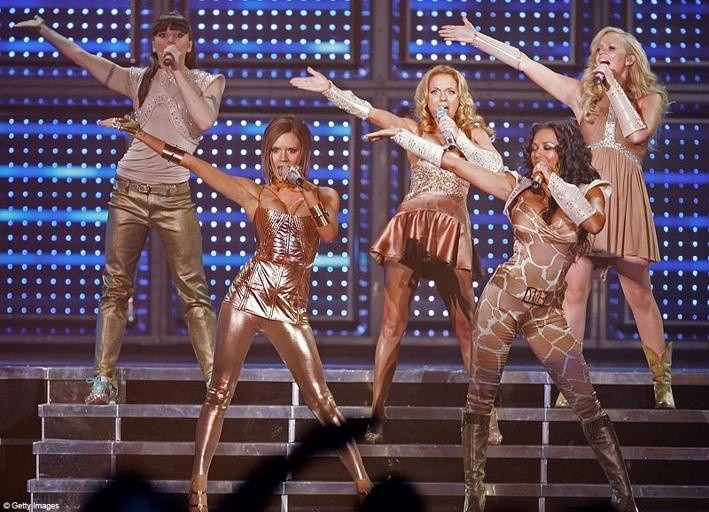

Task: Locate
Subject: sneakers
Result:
[84,381,111,405]
[555,391,572,408]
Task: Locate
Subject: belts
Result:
[113,179,191,197]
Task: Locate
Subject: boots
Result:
[642,340,677,410]
[459,410,492,511]
[582,413,640,512]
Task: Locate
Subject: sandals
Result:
[487,407,503,445]
[364,415,388,444]
[187,487,209,512]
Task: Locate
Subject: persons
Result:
[363,119,639,511]
[439,16,676,410]
[12,10,220,406]
[99,114,374,510]
[291,64,503,445]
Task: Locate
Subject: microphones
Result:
[163,53,174,66]
[593,72,604,85]
[288,172,304,186]
[531,170,543,190]
[435,106,457,150]
